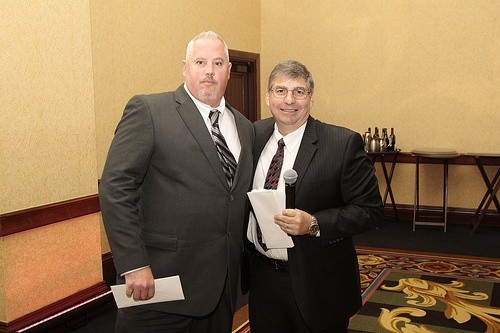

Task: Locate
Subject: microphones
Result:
[283,169,298,236]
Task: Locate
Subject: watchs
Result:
[308,216,319,236]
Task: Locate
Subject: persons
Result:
[98,30,255,333]
[241,60,384,333]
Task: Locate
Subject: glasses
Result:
[269,86,312,100]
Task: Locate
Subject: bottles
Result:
[363,127,395,152]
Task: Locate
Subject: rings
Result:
[287,223,289,228]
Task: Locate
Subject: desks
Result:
[462,152,500,232]
[411,148,460,232]
[364,148,401,228]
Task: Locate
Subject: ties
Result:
[209,110,237,188]
[256,138,285,253]
